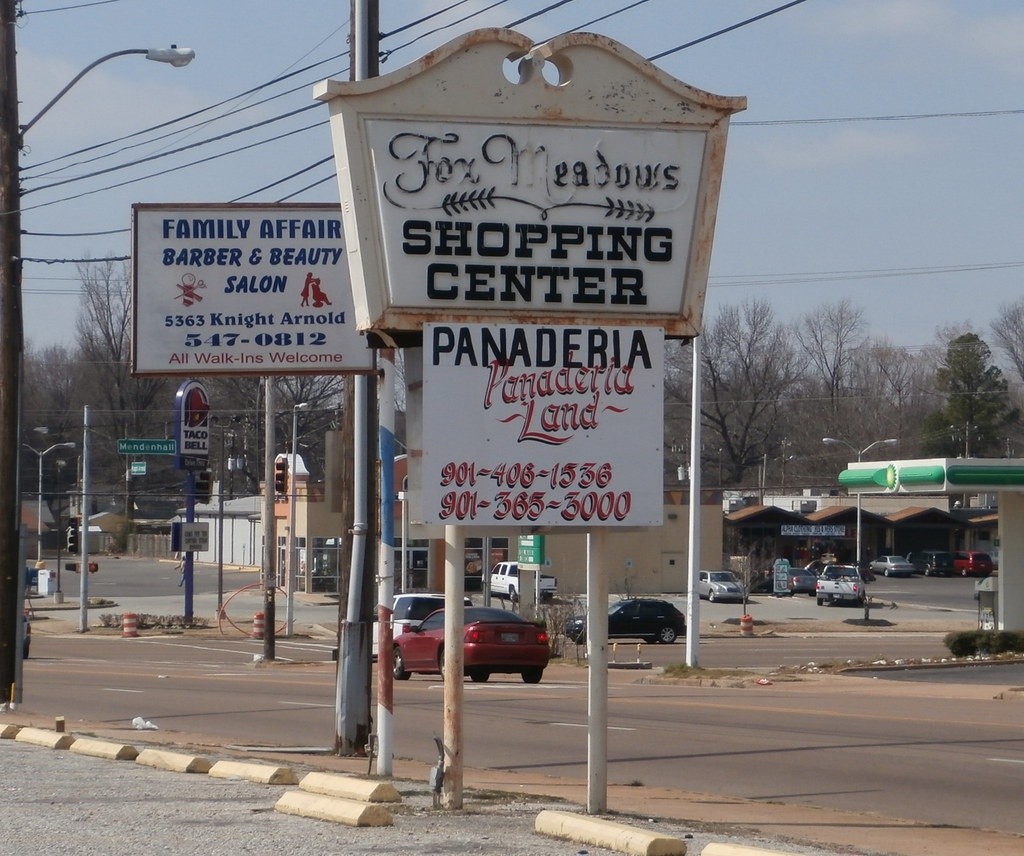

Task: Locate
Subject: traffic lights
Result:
[275,458,287,494]
[67,516,80,556]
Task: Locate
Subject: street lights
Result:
[20,442,76,570]
[822,437,899,566]
[0,44,196,696]
[285,402,311,635]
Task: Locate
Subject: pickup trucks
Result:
[815,564,866,607]
[490,562,559,603]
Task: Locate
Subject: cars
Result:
[392,604,551,686]
[697,569,749,603]
[788,567,817,596]
[952,549,993,577]
[869,556,917,577]
[371,593,475,666]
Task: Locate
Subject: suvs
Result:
[559,596,686,646]
[914,549,954,577]
